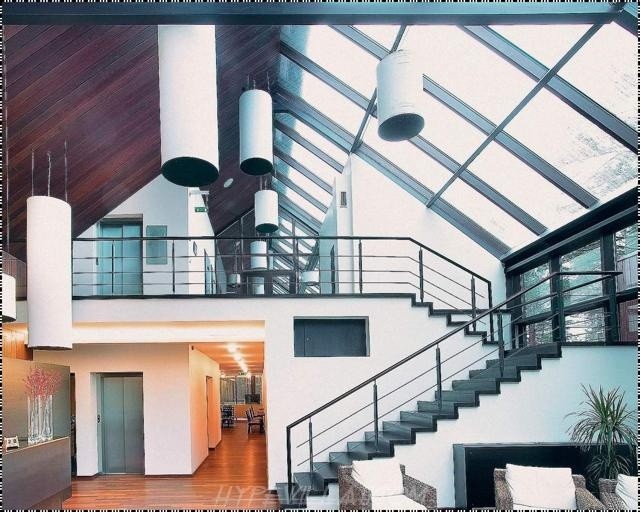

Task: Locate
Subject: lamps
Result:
[158,24,222,189]
[26,139,72,351]
[374,46,424,143]
[253,183,280,233]
[2,272,17,322]
[222,238,322,295]
[238,75,274,177]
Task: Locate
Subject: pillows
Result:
[351,460,405,496]
[615,473,638,510]
[505,462,576,509]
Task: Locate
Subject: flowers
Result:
[21,365,62,432]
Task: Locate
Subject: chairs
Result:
[598,478,638,510]
[246,404,264,433]
[220,405,234,429]
[338,464,437,510]
[493,468,605,509]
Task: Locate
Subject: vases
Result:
[27,395,54,446]
